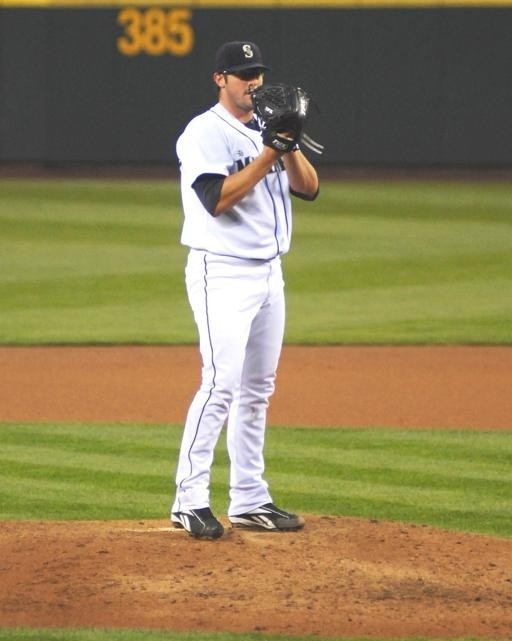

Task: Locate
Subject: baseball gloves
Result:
[250,83,311,152]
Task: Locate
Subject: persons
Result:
[168,37,321,541]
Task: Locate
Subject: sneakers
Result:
[170,505,223,541]
[228,504,305,532]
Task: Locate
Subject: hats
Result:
[214,40,270,74]
